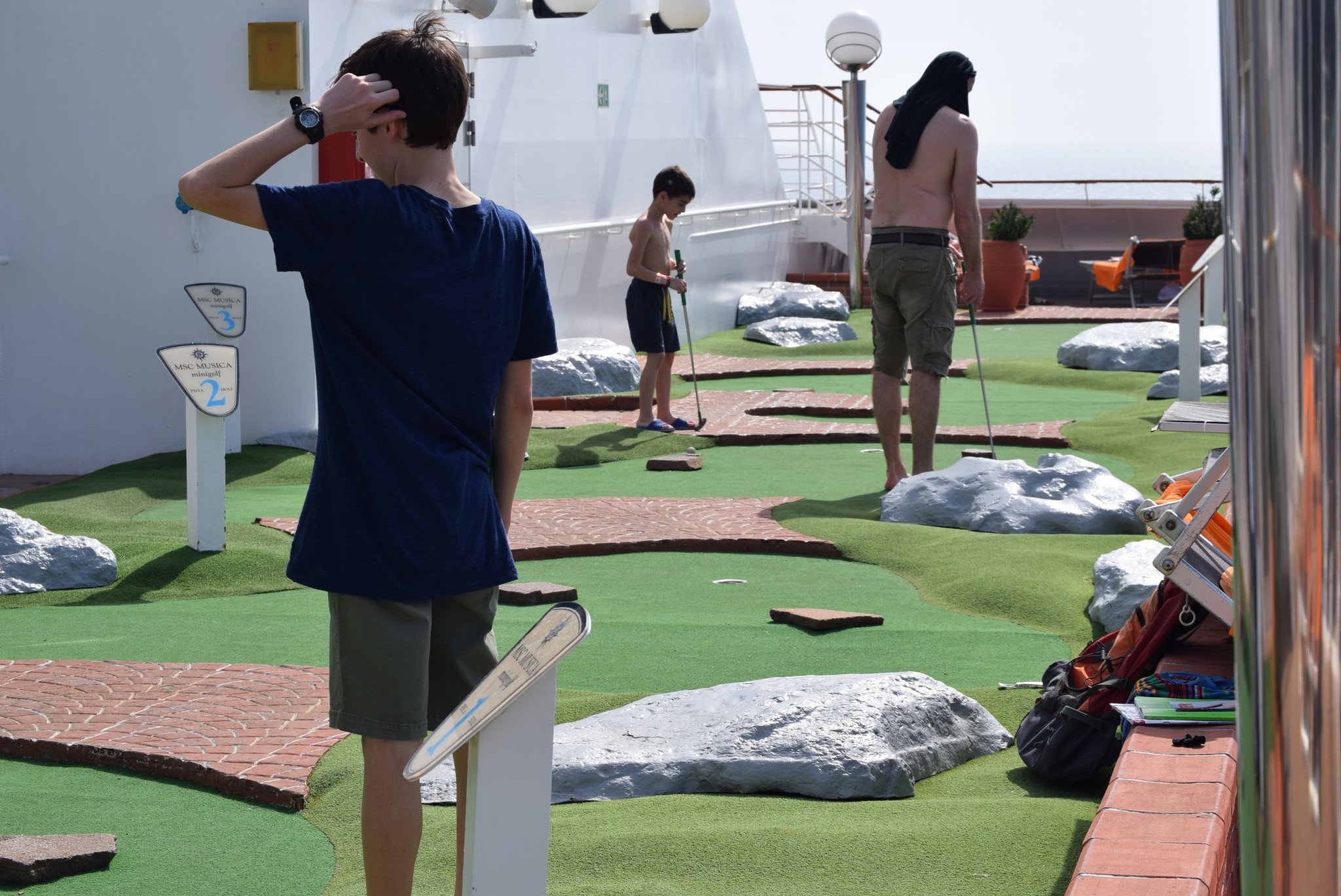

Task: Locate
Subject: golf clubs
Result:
[673,248,706,432]
[961,260,996,460]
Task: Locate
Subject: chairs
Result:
[1079,236,1187,308]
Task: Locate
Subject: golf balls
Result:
[687,447,696,454]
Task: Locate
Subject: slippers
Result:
[671,417,695,429]
[636,419,675,431]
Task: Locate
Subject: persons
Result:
[625,165,696,432]
[177,9,559,896]
[864,52,985,491]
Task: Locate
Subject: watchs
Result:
[290,96,325,145]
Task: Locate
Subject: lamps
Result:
[440,0,497,19]
[650,0,711,34]
[532,0,599,19]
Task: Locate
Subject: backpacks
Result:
[1013,575,1210,777]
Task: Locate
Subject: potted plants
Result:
[981,202,1035,312]
[1179,186,1224,311]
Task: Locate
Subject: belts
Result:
[870,232,950,247]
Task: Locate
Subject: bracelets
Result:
[654,272,661,284]
[665,275,671,288]
